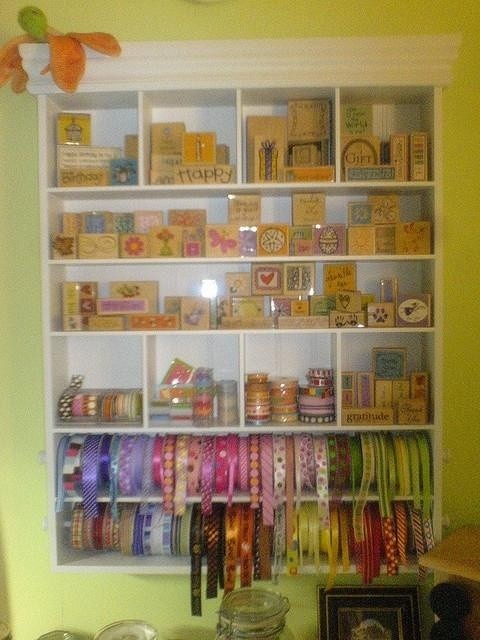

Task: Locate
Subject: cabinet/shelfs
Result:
[40,87,442,575]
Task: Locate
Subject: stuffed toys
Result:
[0,6,123,94]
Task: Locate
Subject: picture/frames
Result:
[316,583,422,640]
